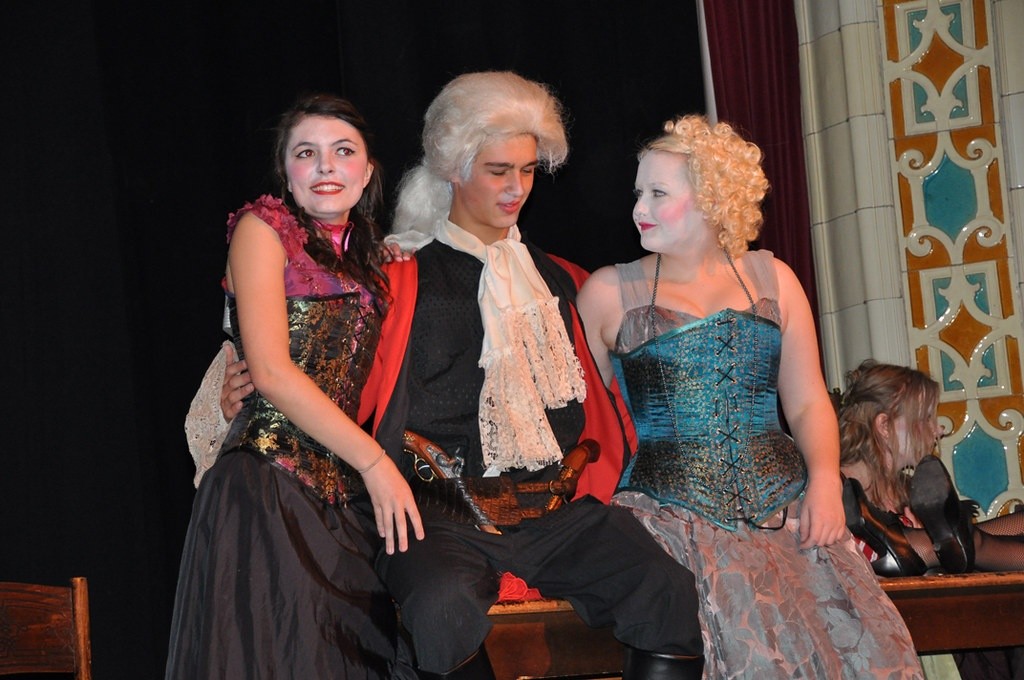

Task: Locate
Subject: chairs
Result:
[0,576,91,680]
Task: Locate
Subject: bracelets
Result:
[357,448,386,473]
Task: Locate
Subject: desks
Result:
[482,570,1024,680]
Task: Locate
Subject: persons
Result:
[165,98,426,678]
[845,455,1023,576]
[579,114,932,679]
[218,70,707,679]
[826,358,1024,680]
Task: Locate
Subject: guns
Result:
[545,438,601,510]
[403,429,502,535]
